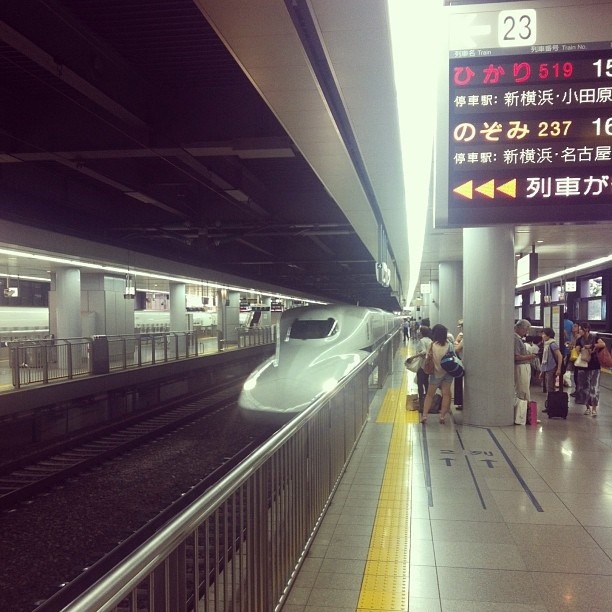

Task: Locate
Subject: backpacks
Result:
[550,343,569,374]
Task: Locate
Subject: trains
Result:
[235,305,413,439]
[0,305,253,348]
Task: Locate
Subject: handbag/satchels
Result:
[527,401,538,426]
[419,343,434,374]
[405,394,418,411]
[597,346,612,367]
[514,396,528,426]
[573,353,589,368]
[422,394,442,414]
[440,343,464,378]
[570,347,578,361]
[581,346,592,362]
[404,355,423,372]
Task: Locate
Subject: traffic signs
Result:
[447,41,611,225]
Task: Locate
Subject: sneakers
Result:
[584,409,592,415]
[592,410,598,415]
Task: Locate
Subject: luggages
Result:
[544,372,568,419]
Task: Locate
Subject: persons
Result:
[514,319,540,425]
[524,336,540,355]
[408,319,463,423]
[576,321,605,417]
[563,312,580,397]
[539,328,563,412]
[402,316,422,341]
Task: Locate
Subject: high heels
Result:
[420,417,427,424]
[440,418,444,424]
